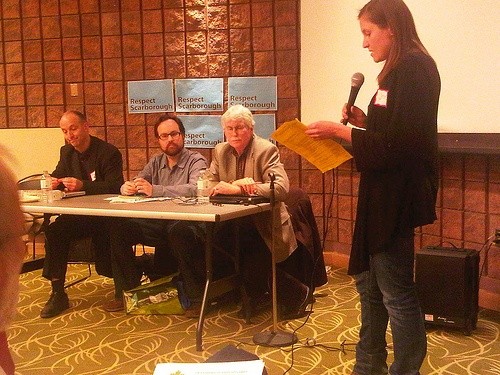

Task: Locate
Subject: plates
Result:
[19,196,40,202]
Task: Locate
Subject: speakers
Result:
[416,246,481,329]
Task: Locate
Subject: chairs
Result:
[17,173,59,260]
[257,190,324,314]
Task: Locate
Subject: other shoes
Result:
[41,293,69,318]
[238,293,272,318]
[186,303,210,318]
[103,298,124,311]
[281,286,312,319]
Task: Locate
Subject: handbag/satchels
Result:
[124,272,191,315]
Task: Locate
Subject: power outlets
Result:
[494,229,500,247]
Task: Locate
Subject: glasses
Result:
[158,132,183,140]
[171,197,198,205]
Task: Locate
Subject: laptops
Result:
[208,194,266,203]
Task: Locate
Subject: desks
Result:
[17,190,274,353]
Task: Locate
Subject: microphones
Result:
[343,71,364,126]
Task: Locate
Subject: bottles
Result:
[50,189,67,200]
[197,170,210,204]
[40,170,53,204]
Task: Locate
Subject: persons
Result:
[105,116,210,319]
[304,0,441,375]
[202,104,311,319]
[40,109,124,319]
